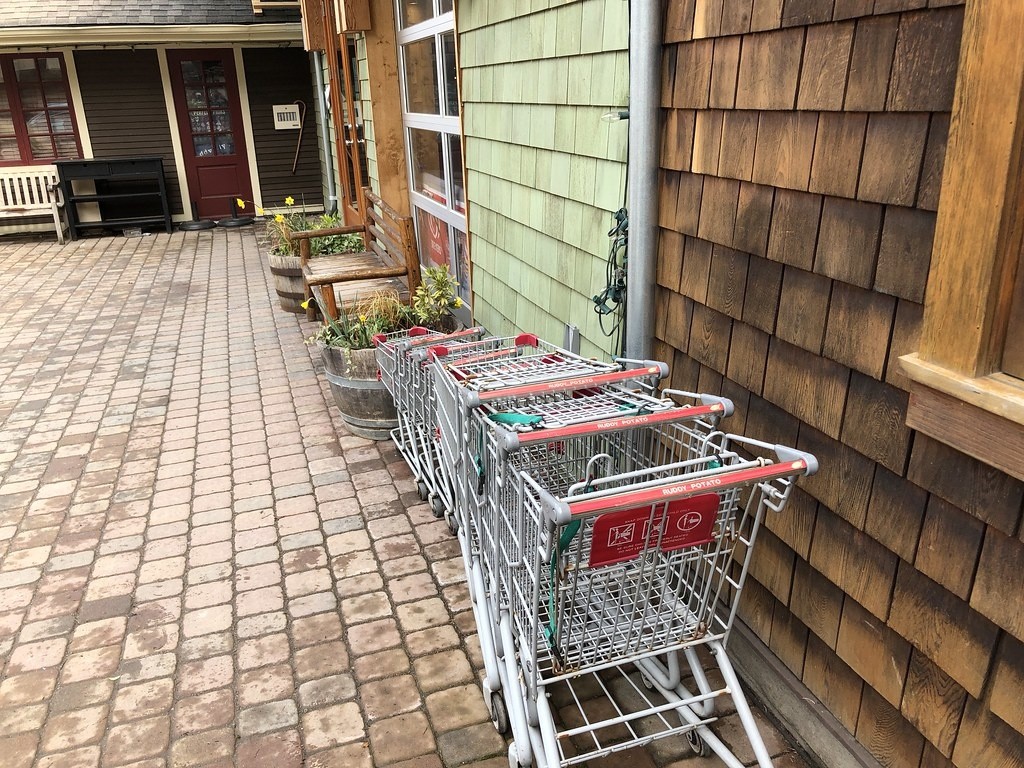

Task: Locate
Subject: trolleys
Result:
[373,322,819,768]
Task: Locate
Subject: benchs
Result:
[289,185,421,327]
[0,165,72,245]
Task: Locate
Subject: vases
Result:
[316,313,463,440]
[267,251,321,314]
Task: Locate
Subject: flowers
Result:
[236,193,366,257]
[301,263,464,380]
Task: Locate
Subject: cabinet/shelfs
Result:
[51,156,173,241]
